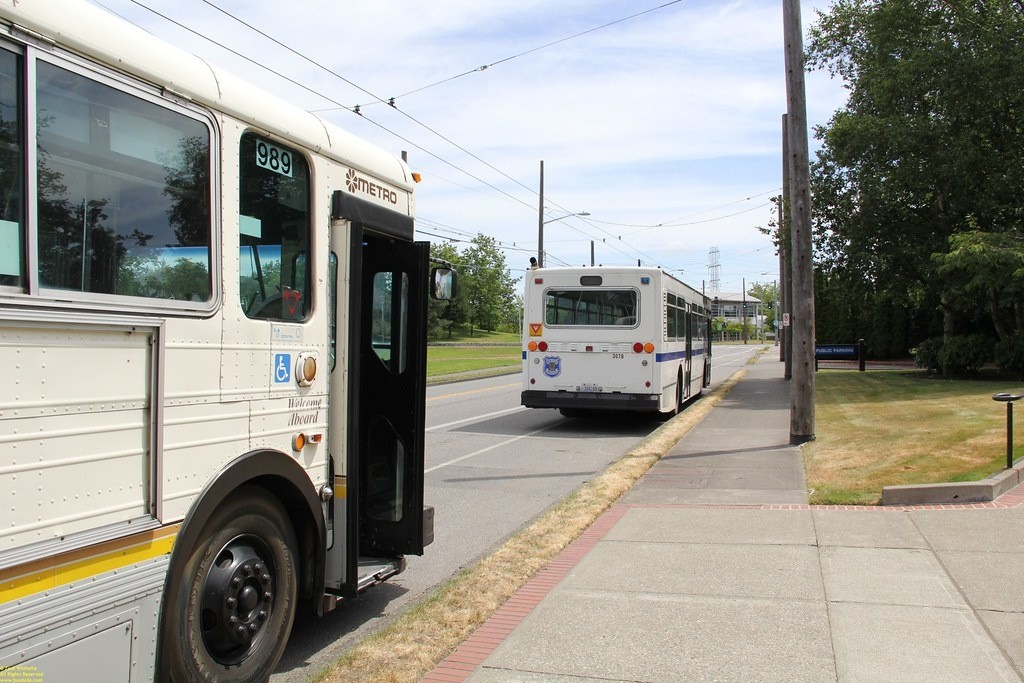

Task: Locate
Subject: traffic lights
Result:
[768,301,772,309]
[743,303,747,309]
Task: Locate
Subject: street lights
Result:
[537,160,590,268]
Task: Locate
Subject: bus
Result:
[0,1,456,683]
[520,266,728,420]
